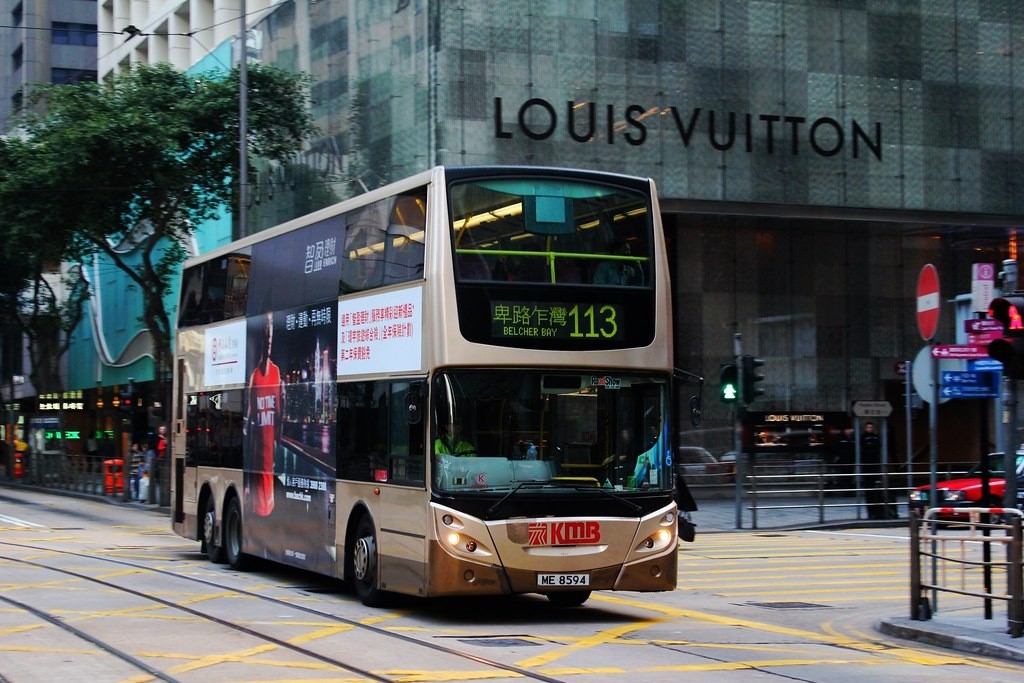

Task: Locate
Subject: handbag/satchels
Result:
[678,513,695,542]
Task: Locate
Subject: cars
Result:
[906,445,1024,530]
[720,450,735,481]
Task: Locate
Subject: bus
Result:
[170,165,704,611]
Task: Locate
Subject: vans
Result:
[678,446,720,483]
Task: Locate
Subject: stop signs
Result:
[916,265,940,339]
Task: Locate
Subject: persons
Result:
[84,430,101,473]
[434,420,475,457]
[594,238,644,287]
[828,422,881,497]
[244,311,281,520]
[130,443,155,504]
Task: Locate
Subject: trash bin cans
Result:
[863,477,898,519]
[104,459,125,493]
[13,453,23,478]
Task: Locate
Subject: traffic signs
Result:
[962,317,1004,331]
[940,371,1002,387]
[966,333,1014,345]
[942,386,1000,398]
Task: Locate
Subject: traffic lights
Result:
[119,390,134,413]
[930,345,991,360]
[719,363,737,405]
[743,353,765,404]
[987,291,1024,381]
